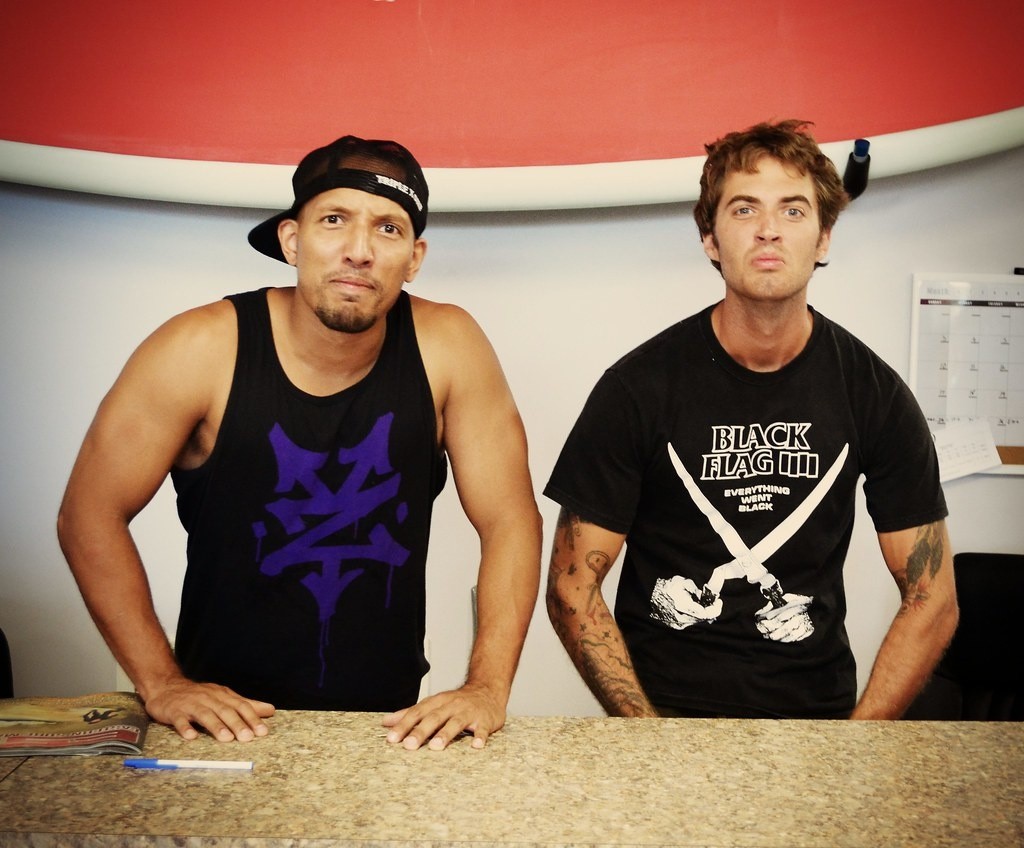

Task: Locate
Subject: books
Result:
[1,691,153,757]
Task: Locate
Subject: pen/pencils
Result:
[123,758,253,772]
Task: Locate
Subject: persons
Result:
[52,137,546,750]
[543,116,963,719]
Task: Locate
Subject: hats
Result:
[247,135,430,268]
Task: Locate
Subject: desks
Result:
[0,703,1024,846]
